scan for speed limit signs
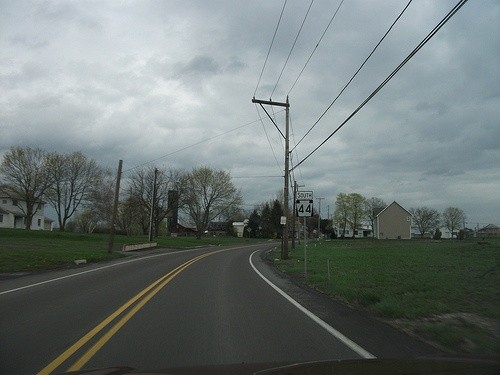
[295,200,313,217]
